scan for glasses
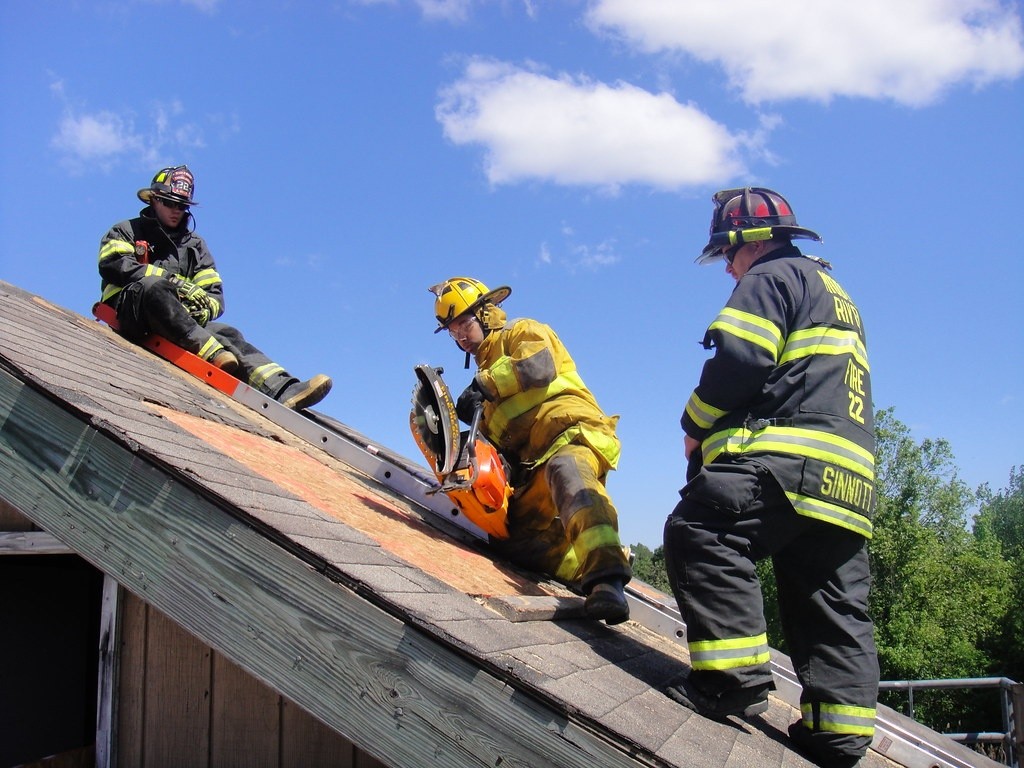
[155,197,190,211]
[449,316,477,341]
[722,240,749,266]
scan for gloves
[456,378,486,427]
[165,271,211,311]
[188,302,211,329]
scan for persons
[661,188,879,768]
[427,276,633,626]
[98,165,332,410]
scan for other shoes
[789,719,860,768]
[666,675,769,721]
[584,575,630,626]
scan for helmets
[428,276,512,335]
[694,186,823,267]
[137,164,199,206]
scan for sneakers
[209,348,239,377]
[276,374,333,413]
